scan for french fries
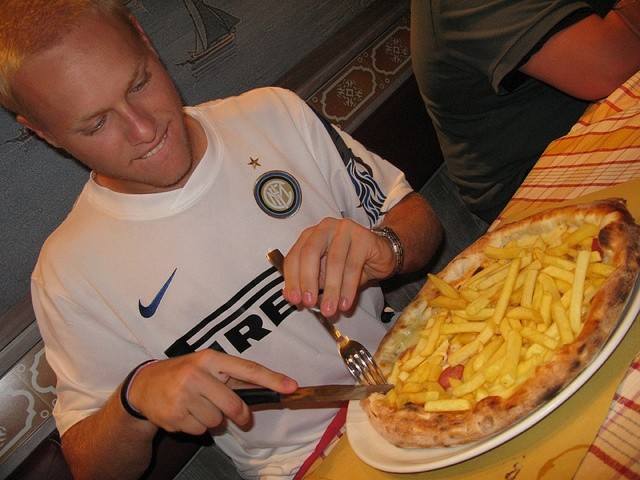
[388,221,611,411]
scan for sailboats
[175,0,242,70]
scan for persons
[0,0,447,478]
[409,1,640,228]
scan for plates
[342,284,639,475]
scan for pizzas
[359,197,637,448]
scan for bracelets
[613,1,640,37]
[368,222,406,280]
[119,356,158,422]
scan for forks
[267,249,387,388]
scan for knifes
[219,384,395,406]
